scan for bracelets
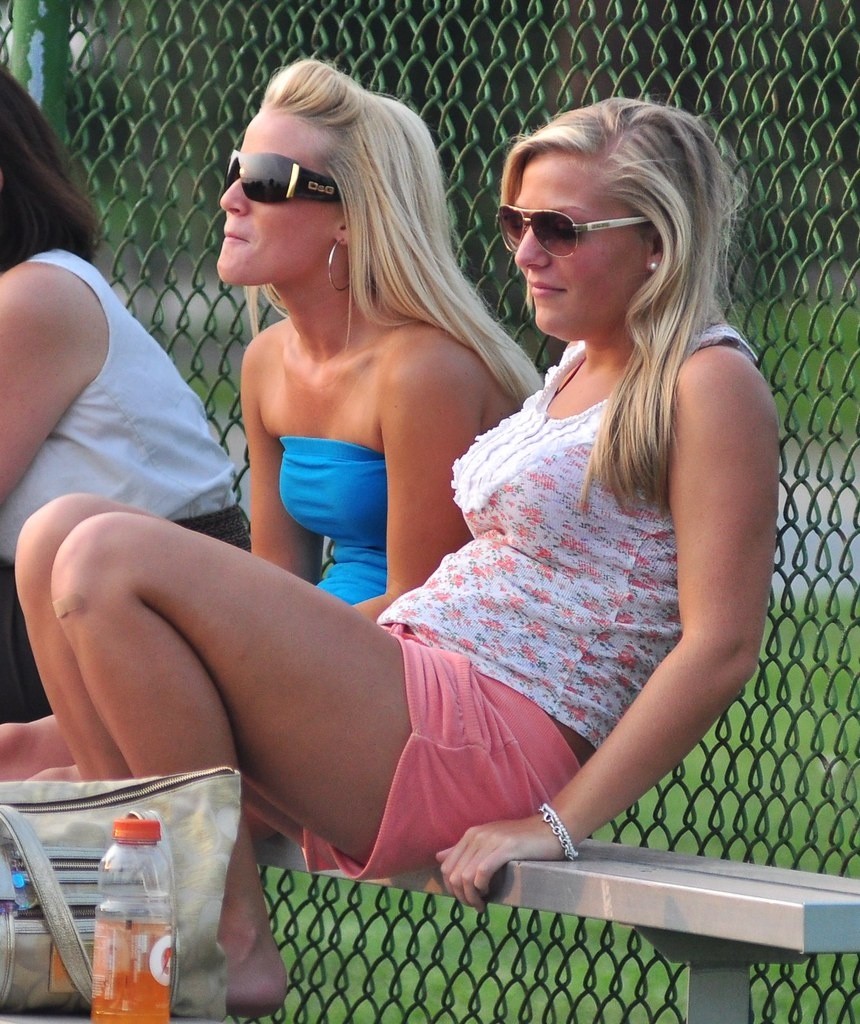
[540,803,579,860]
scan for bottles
[90,818,176,1024]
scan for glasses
[223,148,341,203]
[495,204,652,258]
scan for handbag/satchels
[0,767,241,1022]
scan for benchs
[253,822,860,1024]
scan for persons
[0,59,540,784]
[1,65,250,726]
[14,98,781,1017]
